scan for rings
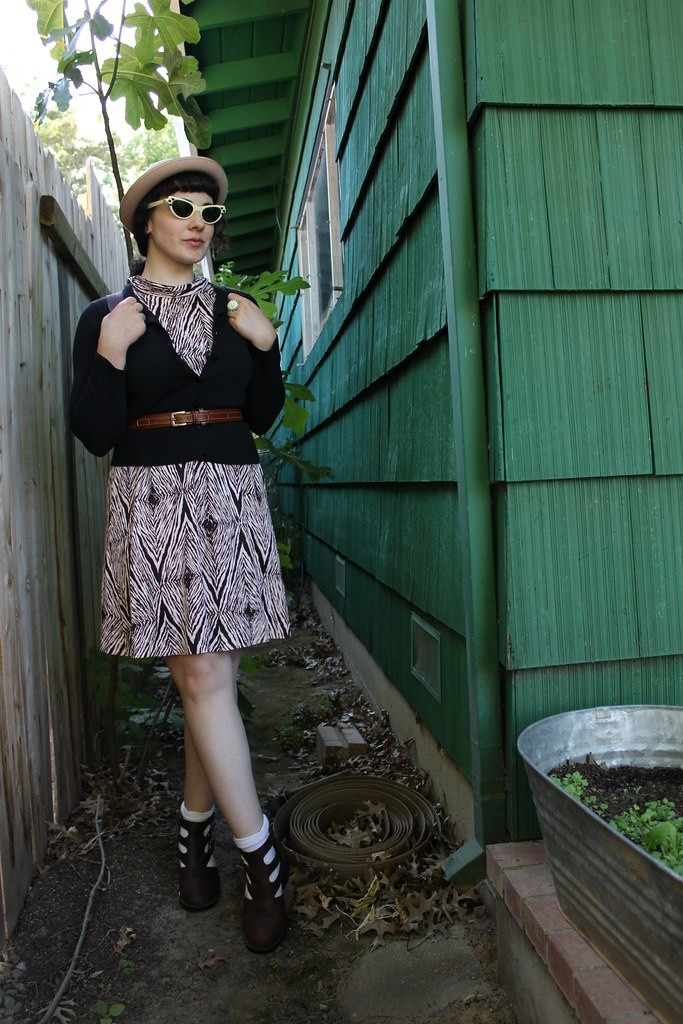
[227,299,239,311]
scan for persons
[69,156,289,950]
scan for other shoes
[235,828,291,953]
[177,813,222,911]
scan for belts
[128,408,245,429]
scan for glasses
[148,195,226,224]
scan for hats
[119,156,227,234]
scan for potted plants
[519,702,683,1024]
